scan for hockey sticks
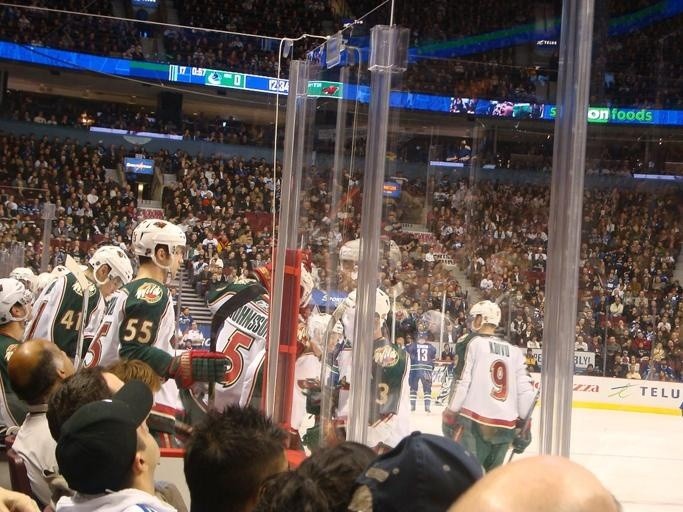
[318,292,356,452]
[61,253,89,370]
[208,285,261,411]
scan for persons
[0,0,682,512]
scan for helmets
[130,218,187,270]
[1,267,35,325]
[89,244,134,286]
[468,300,502,331]
[299,238,390,353]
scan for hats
[54,380,154,495]
[341,431,484,512]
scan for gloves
[176,350,229,388]
[252,248,312,292]
[441,408,462,437]
[512,419,533,454]
